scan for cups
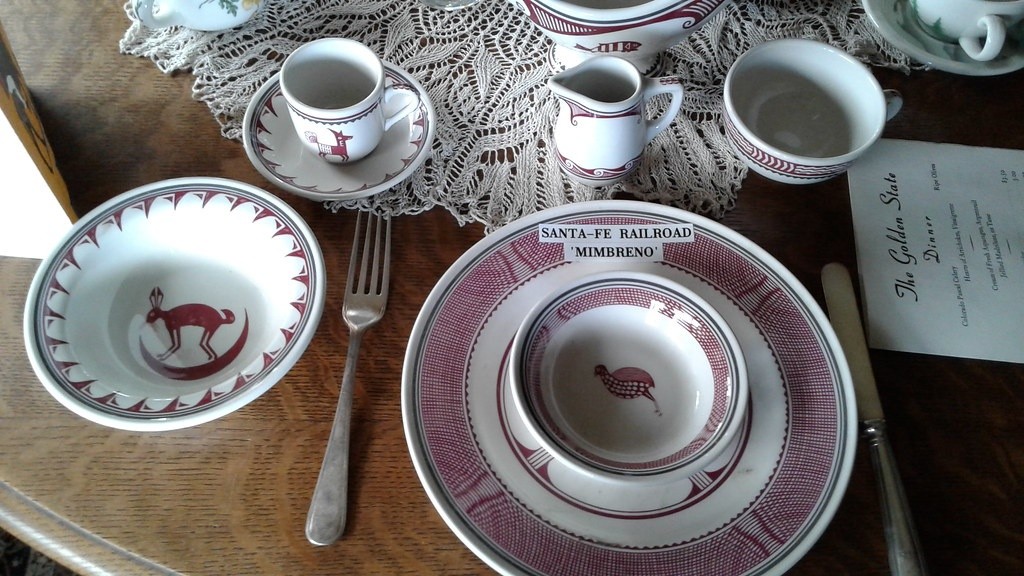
[725,38,886,184]
[901,0,1024,61]
[279,37,421,163]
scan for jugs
[548,52,684,186]
[508,0,730,92]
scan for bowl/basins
[509,268,751,483]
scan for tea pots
[132,0,271,30]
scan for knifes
[819,261,934,575]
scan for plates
[22,174,329,433]
[239,57,435,199]
[861,0,1024,75]
[401,199,858,576]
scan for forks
[301,207,392,546]
